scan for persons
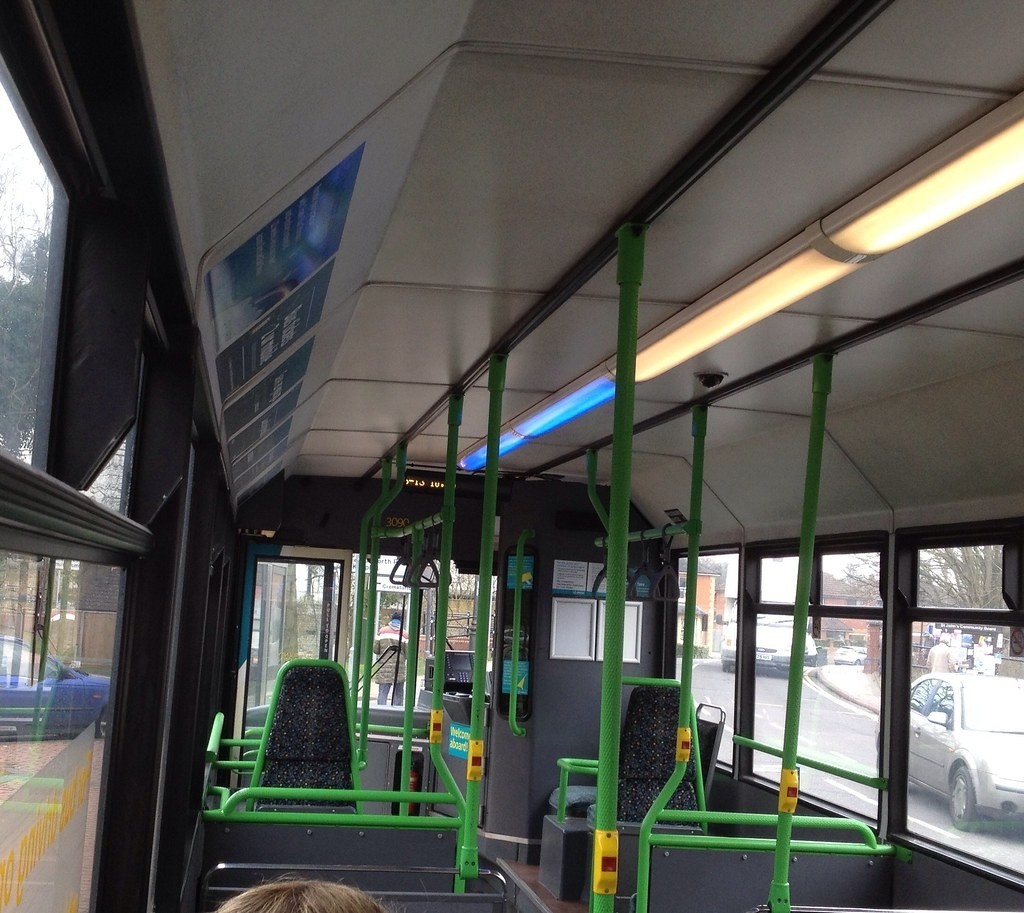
[212,870,417,913]
[373,610,409,706]
[925,632,960,673]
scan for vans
[716,601,793,678]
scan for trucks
[246,574,283,680]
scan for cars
[803,632,822,666]
[0,634,111,741]
[834,646,868,666]
[876,672,1024,832]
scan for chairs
[587,683,709,834]
[547,703,725,815]
[245,658,365,815]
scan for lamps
[452,88,1024,470]
